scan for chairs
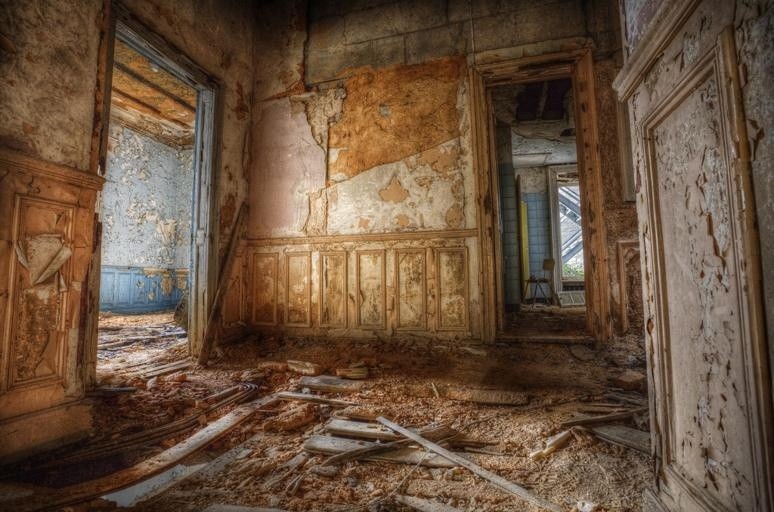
[524,257,562,308]
